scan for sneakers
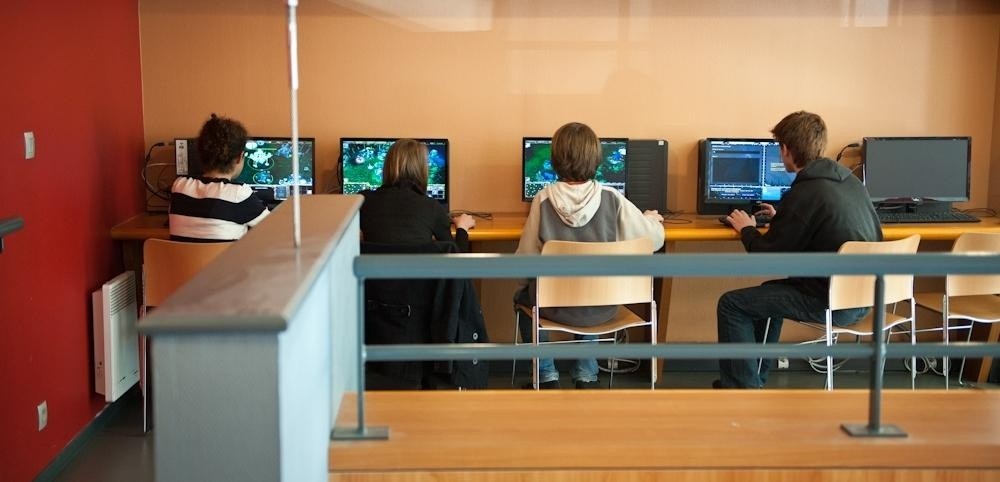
[713,379,722,388]
[522,379,562,388]
[574,375,602,389]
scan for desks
[114,207,1000,380]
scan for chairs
[754,233,920,389]
[511,239,658,389]
[911,231,1000,390]
[141,239,237,434]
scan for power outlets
[37,400,48,431]
[24,132,35,159]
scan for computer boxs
[628,138,668,214]
[697,140,750,215]
[174,137,200,177]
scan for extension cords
[607,360,620,370]
[779,358,789,369]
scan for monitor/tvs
[232,137,314,202]
[703,137,796,214]
[341,137,450,215]
[862,136,973,213]
[522,136,629,200]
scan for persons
[713,109,883,388]
[169,112,271,243]
[357,138,492,389]
[513,121,665,389]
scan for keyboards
[877,213,982,223]
[718,216,772,228]
